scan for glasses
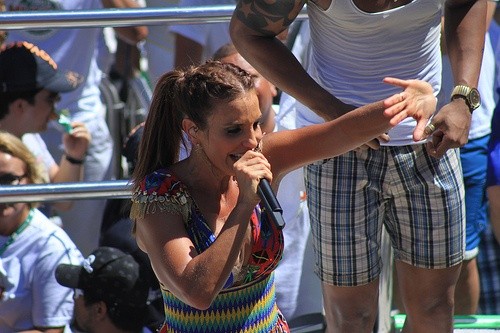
[0,172,28,185]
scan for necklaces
[198,149,233,216]
[0,208,34,257]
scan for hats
[55,246,149,306]
[0,41,84,91]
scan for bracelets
[64,149,87,165]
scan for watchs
[450,85,481,110]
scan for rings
[427,123,436,132]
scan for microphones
[257,178,286,230]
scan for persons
[55,247,148,332]
[441,0,500,315]
[0,0,149,259]
[100,122,167,333]
[0,132,85,333]
[229,0,488,333]
[211,43,323,331]
[0,41,91,228]
[166,0,289,72]
[121,60,437,333]
